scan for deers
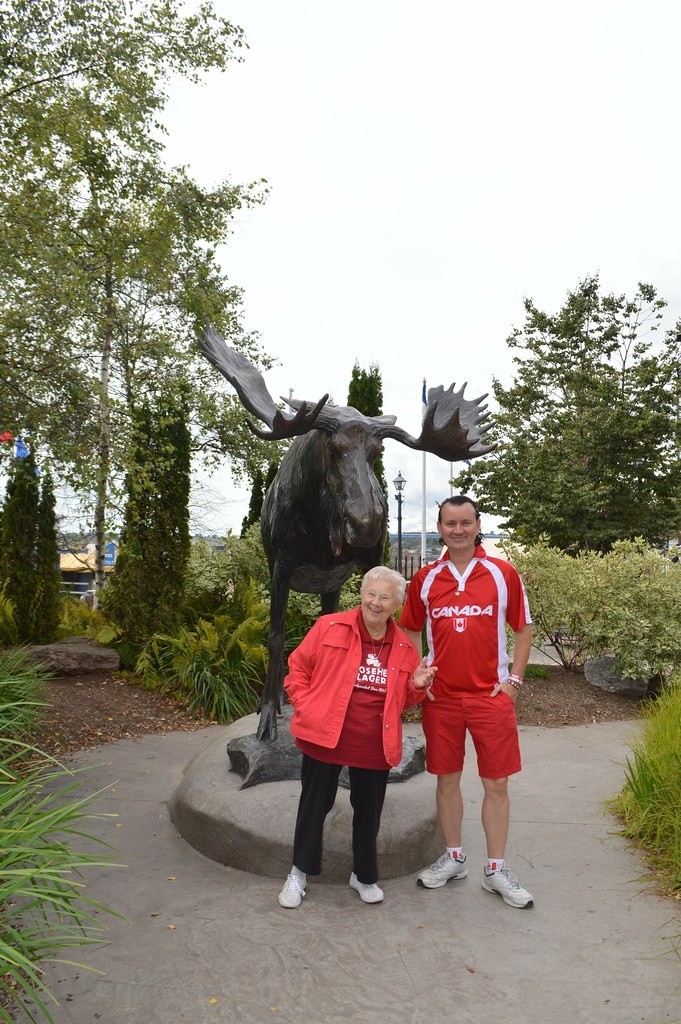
[192,318,500,741]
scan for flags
[464,460,471,466]
[422,383,428,416]
[17,436,40,475]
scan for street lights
[393,470,406,574]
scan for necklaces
[372,637,384,659]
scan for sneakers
[481,866,535,910]
[349,871,385,904]
[417,853,470,889]
[278,873,308,909]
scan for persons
[399,495,534,909]
[507,673,524,690]
[278,565,438,908]
